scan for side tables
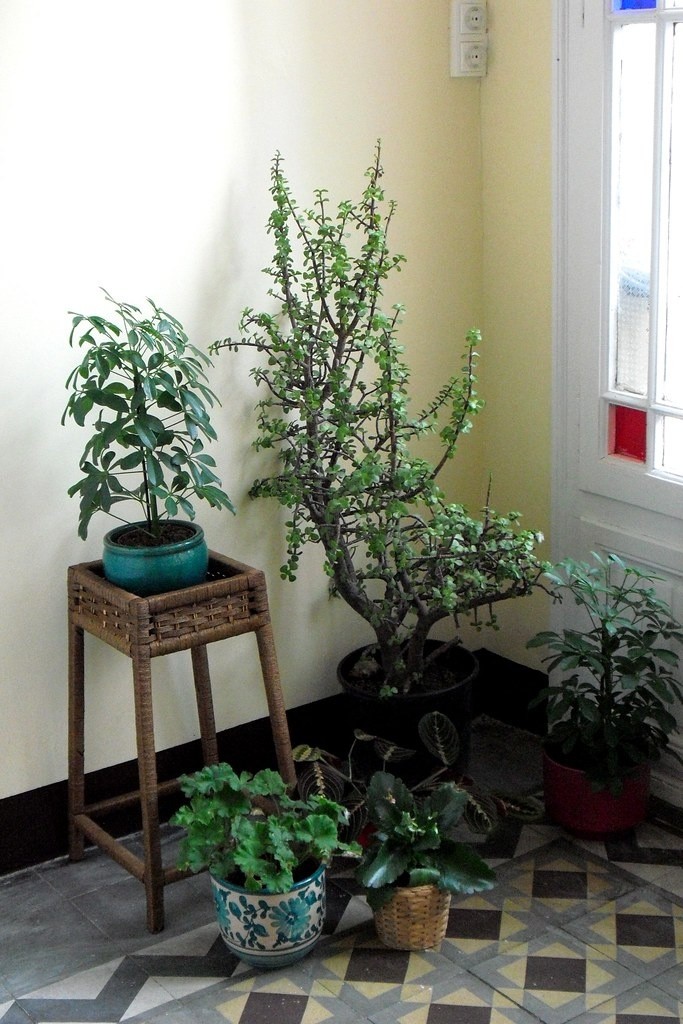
[67,552,301,928]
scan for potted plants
[209,136,566,782]
[354,774,500,950]
[57,278,236,593]
[528,547,683,830]
[171,763,361,968]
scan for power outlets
[450,1,488,81]
